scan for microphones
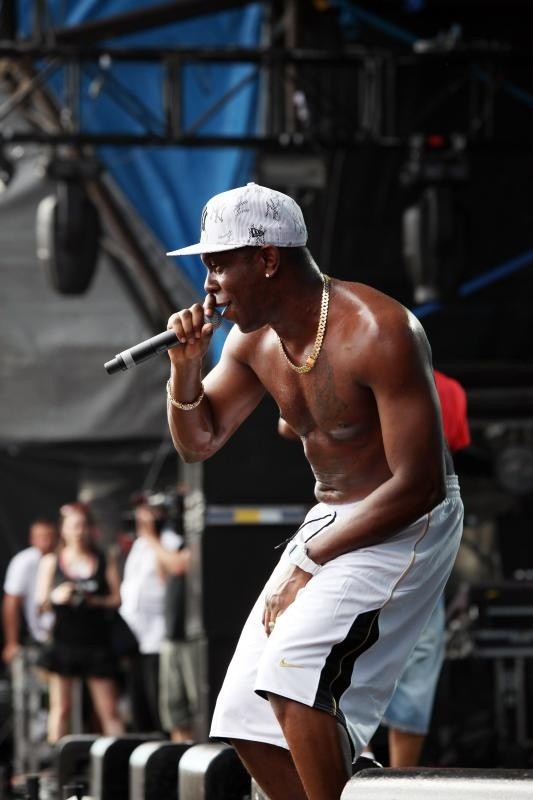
[103,309,223,378]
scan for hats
[165,181,308,256]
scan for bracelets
[166,378,204,411]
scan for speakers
[182,391,318,747]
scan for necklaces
[276,274,330,374]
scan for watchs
[287,542,322,575]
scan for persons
[0,490,195,747]
[165,182,464,800]
[346,328,470,774]
